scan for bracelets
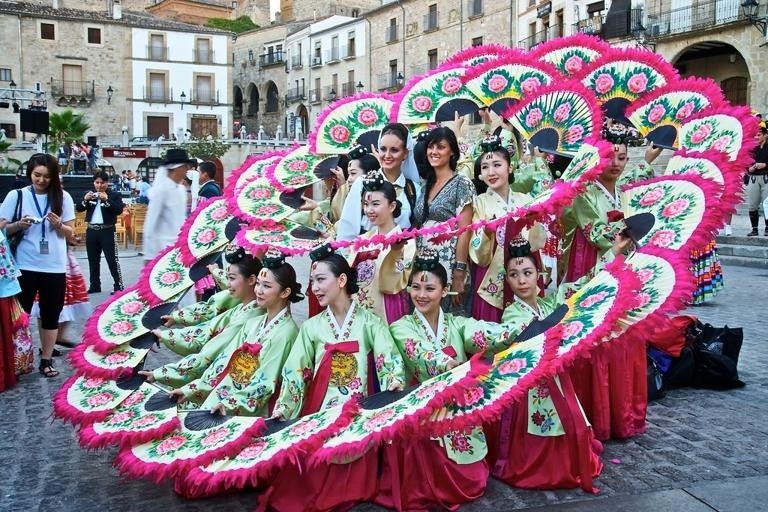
[56,222,63,230]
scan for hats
[160,148,198,166]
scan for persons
[335,172,417,325]
[160,262,244,326]
[755,114,765,127]
[0,152,76,376]
[164,259,299,499]
[156,127,258,141]
[272,244,406,512]
[138,243,261,391]
[76,170,124,293]
[57,143,98,180]
[335,124,422,259]
[286,155,349,225]
[411,128,472,314]
[747,126,768,238]
[724,210,732,236]
[119,169,152,206]
[389,246,538,512]
[299,155,380,224]
[568,132,672,444]
[189,160,223,201]
[502,227,634,489]
[141,150,191,264]
[467,136,569,324]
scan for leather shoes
[39,349,62,358]
[746,229,755,240]
[111,290,119,294]
[86,288,103,294]
[57,339,78,349]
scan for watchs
[454,261,467,271]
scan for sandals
[39,359,60,377]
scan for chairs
[115,215,127,247]
[74,210,87,243]
[130,206,147,250]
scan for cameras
[24,213,47,225]
[91,192,99,198]
